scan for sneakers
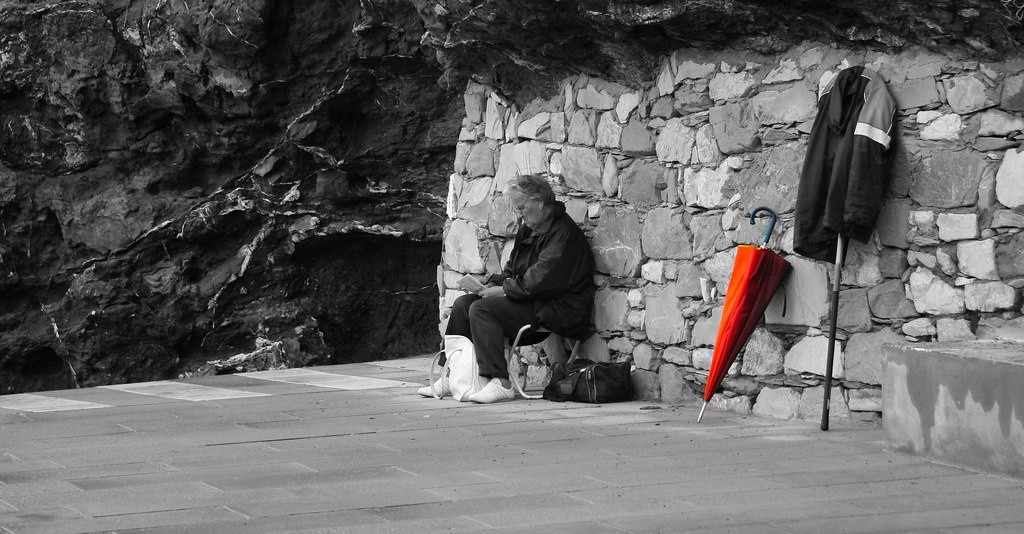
[469,378,515,404]
[418,376,451,397]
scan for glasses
[513,199,528,216]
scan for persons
[417,174,595,404]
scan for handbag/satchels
[429,335,491,401]
[543,357,635,404]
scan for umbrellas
[695,205,794,425]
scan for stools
[503,323,580,399]
